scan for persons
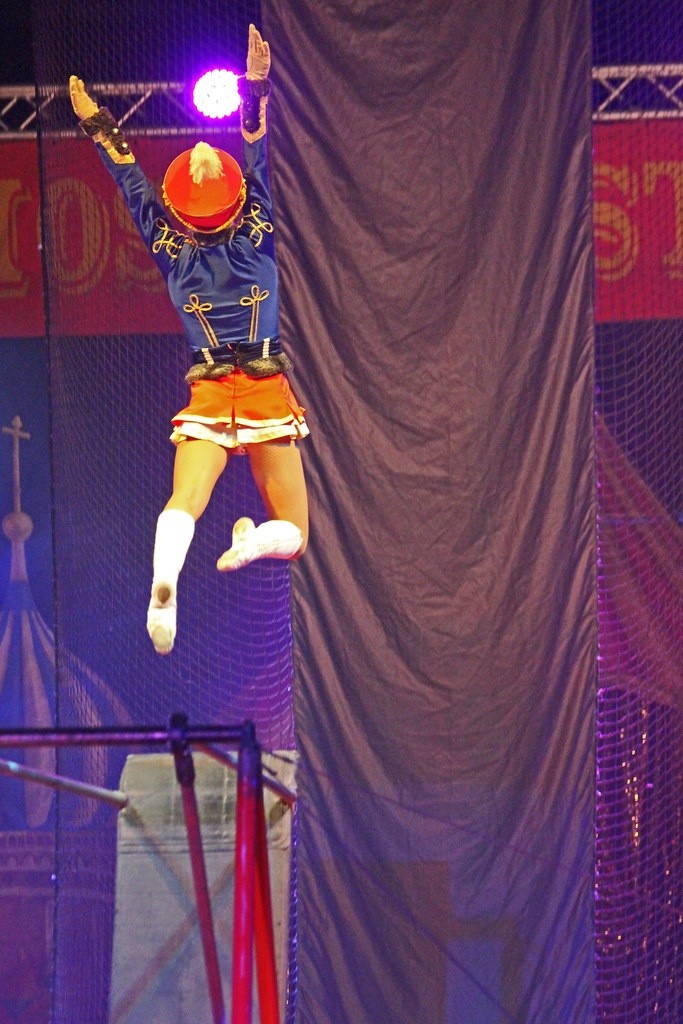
[70,23,310,654]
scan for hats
[163,142,245,235]
